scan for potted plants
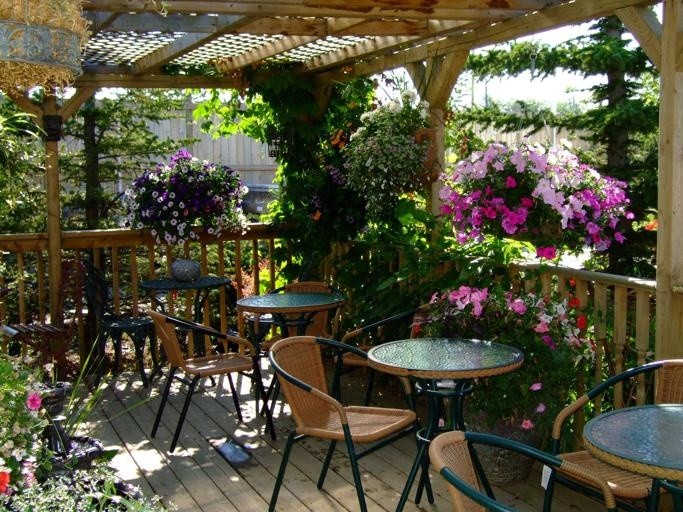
[40,336,157,477]
[0,472,179,512]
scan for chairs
[8,260,83,380]
[429,430,616,512]
[255,281,344,435]
[270,336,435,512]
[85,267,163,388]
[148,309,276,453]
[334,303,433,411]
[542,359,683,512]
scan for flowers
[344,90,432,223]
[125,150,248,247]
[438,141,634,258]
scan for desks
[139,276,230,390]
[583,403,683,512]
[237,293,344,433]
[368,337,523,512]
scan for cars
[241,183,285,222]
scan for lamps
[0,0,93,98]
